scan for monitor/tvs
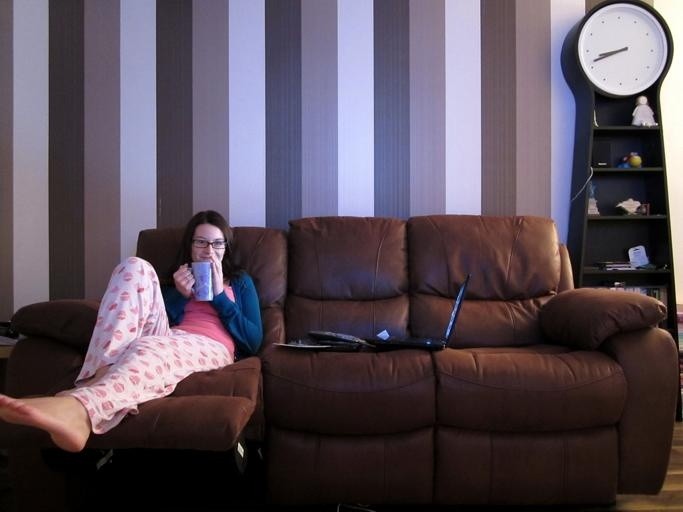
[597,259,635,270]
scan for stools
[33,356,264,512]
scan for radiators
[319,340,360,350]
[308,330,360,343]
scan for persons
[1,210,263,452]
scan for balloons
[577,2,669,99]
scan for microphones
[33,356,264,512]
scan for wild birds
[376,280,467,351]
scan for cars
[184,261,214,302]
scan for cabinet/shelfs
[561,0,683,422]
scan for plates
[561,0,683,422]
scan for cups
[192,239,228,250]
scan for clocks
[577,2,669,99]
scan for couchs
[0,216,680,512]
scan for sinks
[0,244,680,512]
